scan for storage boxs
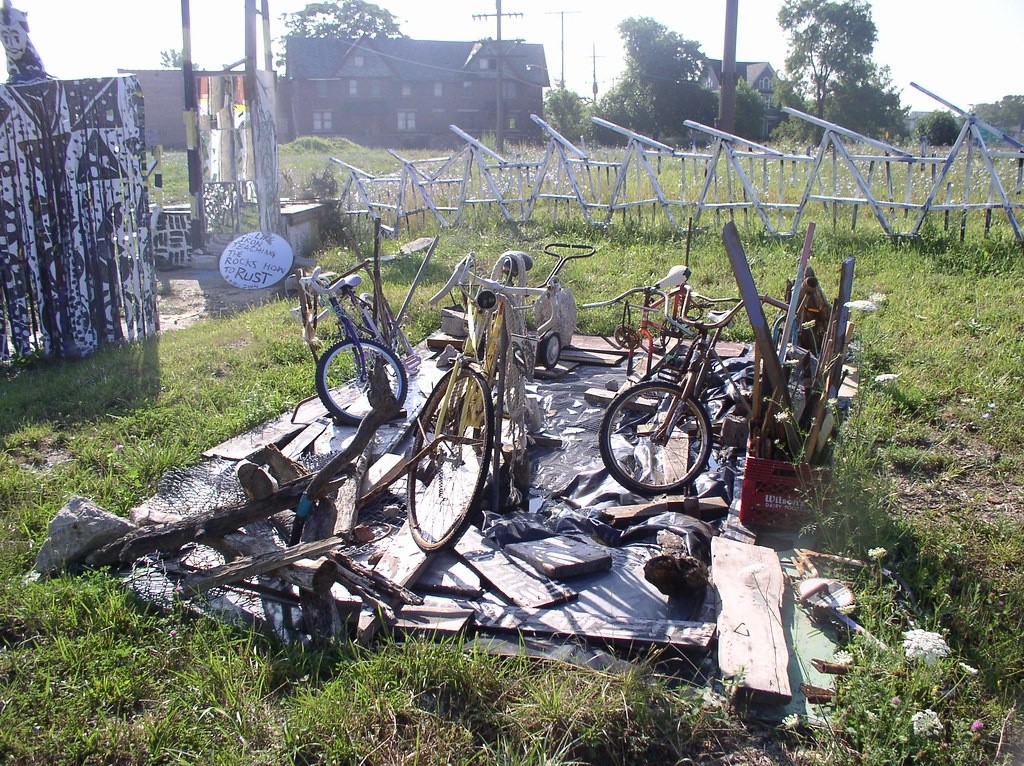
[741,440,832,530]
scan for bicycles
[298,267,422,427]
[405,248,560,553]
[599,284,789,494]
